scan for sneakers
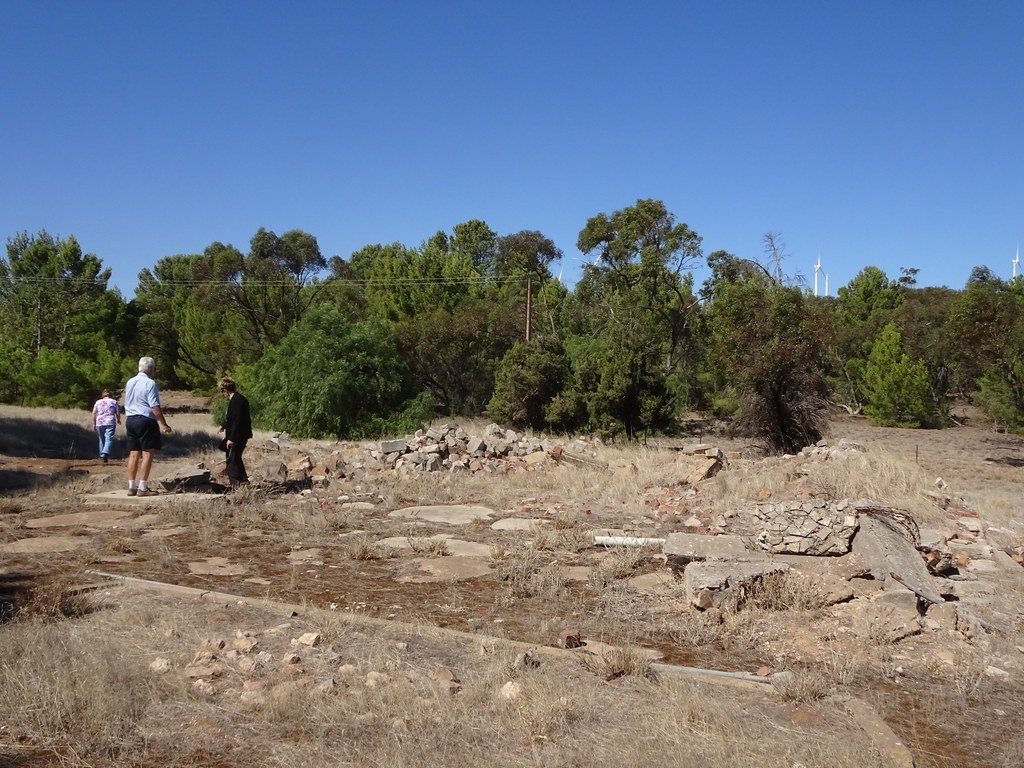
[137,487,159,497]
[127,488,138,496]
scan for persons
[216,377,253,487]
[93,389,123,464]
[124,357,173,497]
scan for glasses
[221,390,224,393]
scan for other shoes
[99,454,108,463]
